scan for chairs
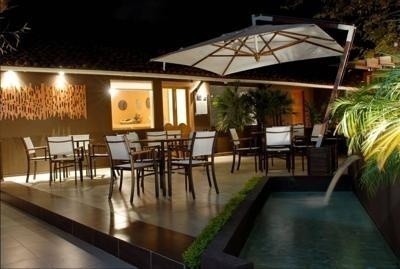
[21,129,219,204]
[229,123,328,175]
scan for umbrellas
[150,15,357,147]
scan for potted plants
[212,79,255,155]
